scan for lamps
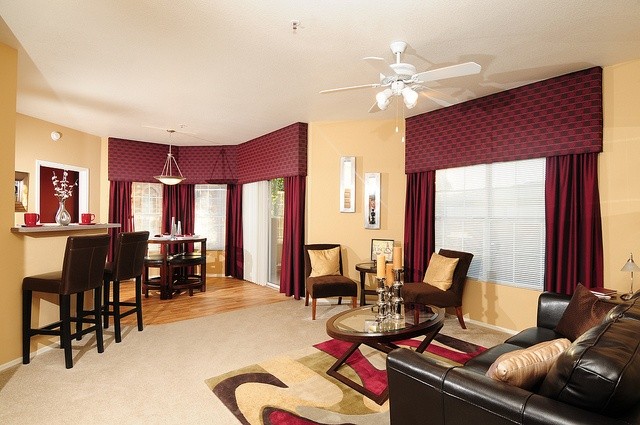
[620,253,640,301]
[153,130,187,186]
[376,80,419,111]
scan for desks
[355,262,407,305]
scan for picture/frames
[371,239,394,261]
[340,156,356,213]
[15,171,29,212]
[363,173,381,230]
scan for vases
[54,202,71,226]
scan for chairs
[103,231,149,343]
[399,249,474,329]
[22,235,111,369]
[304,244,357,320]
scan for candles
[386,264,394,280]
[376,251,386,278]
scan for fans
[320,42,483,115]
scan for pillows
[307,246,341,278]
[422,252,460,292]
[484,338,572,389]
[552,282,617,341]
[600,296,640,323]
[537,319,639,416]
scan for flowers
[51,169,79,202]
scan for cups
[81,214,95,223]
[24,213,39,225]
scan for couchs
[386,293,639,425]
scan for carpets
[203,331,488,424]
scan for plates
[22,225,42,227]
[79,223,96,225]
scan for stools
[144,253,205,300]
